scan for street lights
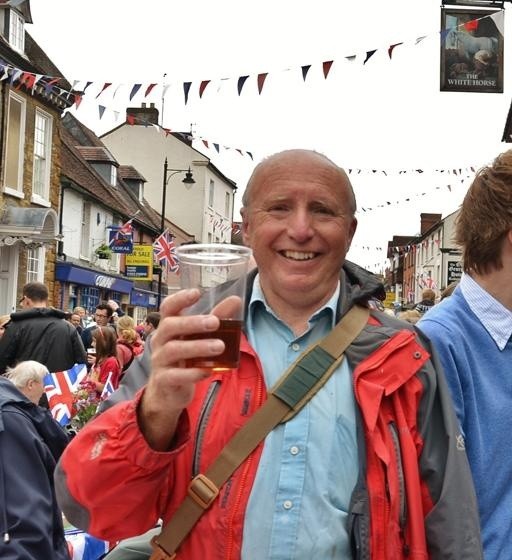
[159,155,196,309]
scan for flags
[113,215,135,241]
[152,229,181,272]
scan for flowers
[72,377,105,424]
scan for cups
[173,241,253,374]
[87,347,97,364]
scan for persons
[414,148,511,559]
[51,147,484,560]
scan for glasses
[19,296,25,306]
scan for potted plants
[94,244,113,260]
